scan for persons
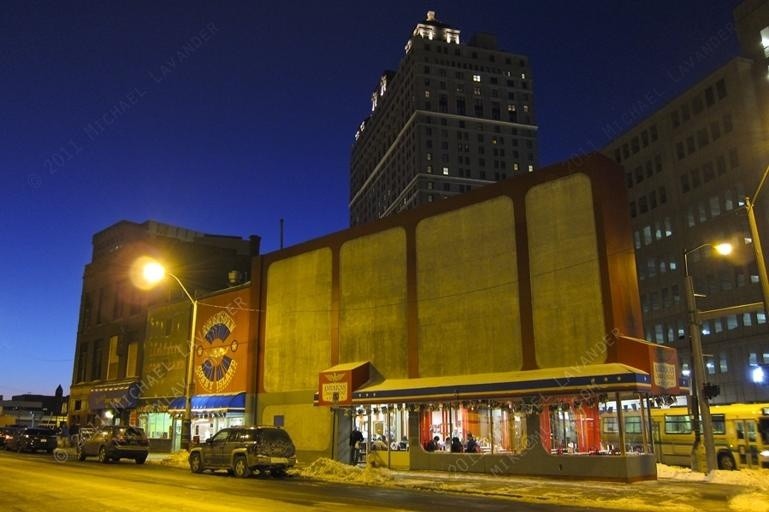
[451,437,462,453]
[372,435,389,450]
[441,438,451,452]
[464,432,477,453]
[348,426,364,466]
[426,436,439,450]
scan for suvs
[188,424,297,477]
[0,420,60,454]
[74,423,151,468]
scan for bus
[599,403,769,473]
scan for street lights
[142,260,198,458]
[682,241,736,474]
